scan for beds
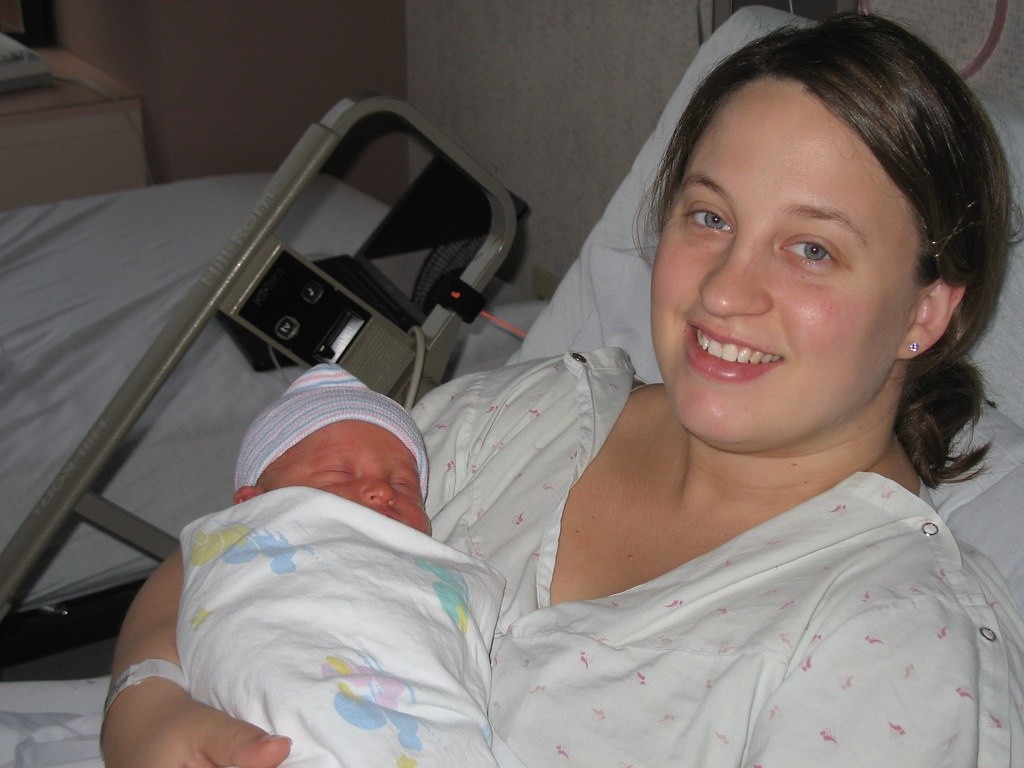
[0,96,549,622]
[0,6,1024,768]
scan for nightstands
[0,47,150,209]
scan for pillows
[570,243,1024,585]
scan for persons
[1,17,1024,768]
[177,360,496,766]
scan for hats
[235,363,428,504]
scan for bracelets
[97,658,189,758]
